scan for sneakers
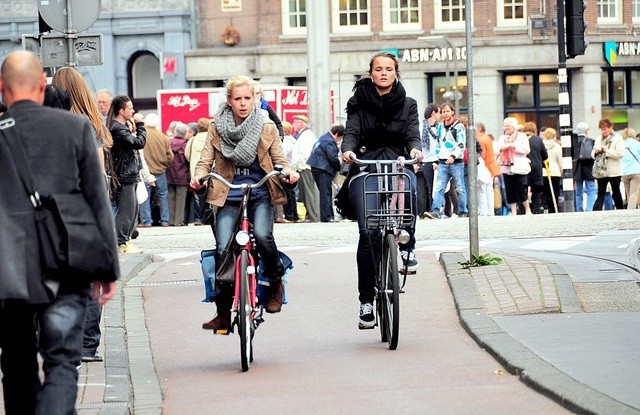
[359,303,376,327]
[424,212,439,219]
[82,350,103,361]
[401,250,418,270]
[120,238,143,254]
[457,213,467,217]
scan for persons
[306,123,345,223]
[635,133,640,142]
[184,116,212,225]
[572,121,596,212]
[538,127,546,138]
[186,122,199,140]
[0,50,120,415]
[139,112,174,227]
[165,123,190,225]
[591,118,625,210]
[474,120,500,216]
[165,120,181,137]
[252,79,284,144]
[494,115,532,216]
[188,75,300,329]
[458,117,482,188]
[106,94,147,254]
[541,126,565,213]
[418,104,442,218]
[49,65,113,362]
[95,89,112,126]
[620,127,640,210]
[39,84,73,112]
[280,120,300,224]
[424,102,467,220]
[521,121,548,214]
[339,50,425,329]
[290,115,320,223]
[124,117,157,241]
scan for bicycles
[191,164,294,371]
[343,153,431,349]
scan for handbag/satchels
[216,226,237,295]
[594,154,608,177]
[494,185,502,209]
[578,138,593,159]
[35,193,118,284]
[201,250,293,304]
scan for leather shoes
[266,278,283,313]
[202,311,231,329]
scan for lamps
[246,54,256,71]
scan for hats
[197,118,209,129]
[293,116,308,125]
